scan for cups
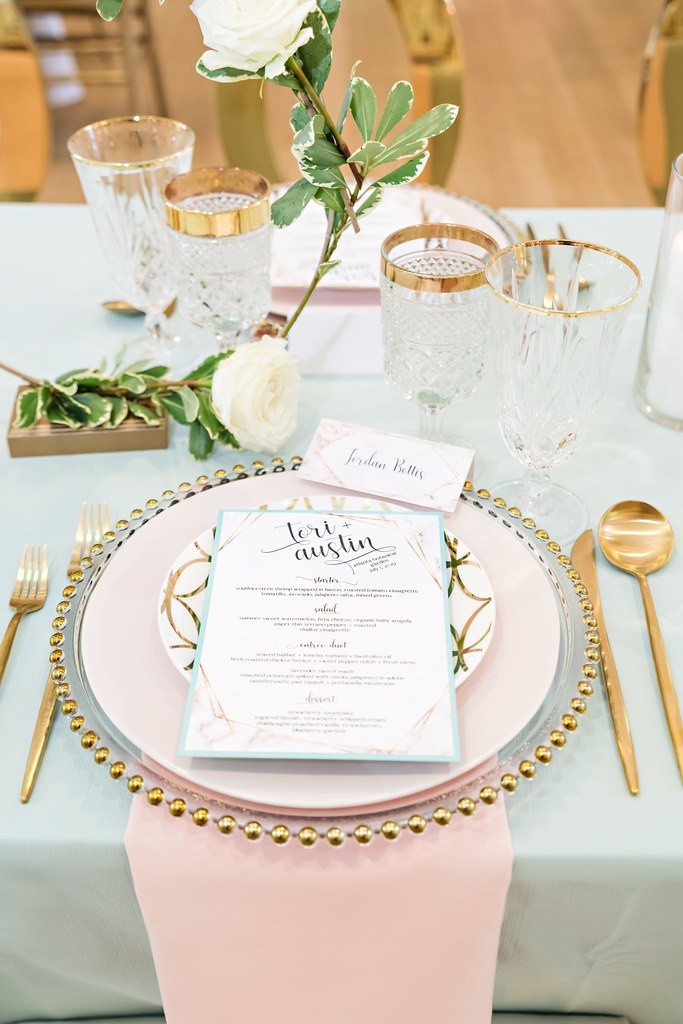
[633,152,683,433]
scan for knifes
[572,530,641,795]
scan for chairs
[2,0,683,210]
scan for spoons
[102,300,143,317]
[599,500,683,785]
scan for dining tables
[0,198,683,1024]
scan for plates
[49,457,601,851]
[270,181,526,299]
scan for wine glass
[485,239,642,546]
[379,223,501,439]
[68,115,196,371]
[158,166,272,352]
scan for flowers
[0,0,471,455]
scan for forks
[0,543,49,682]
[21,500,111,802]
